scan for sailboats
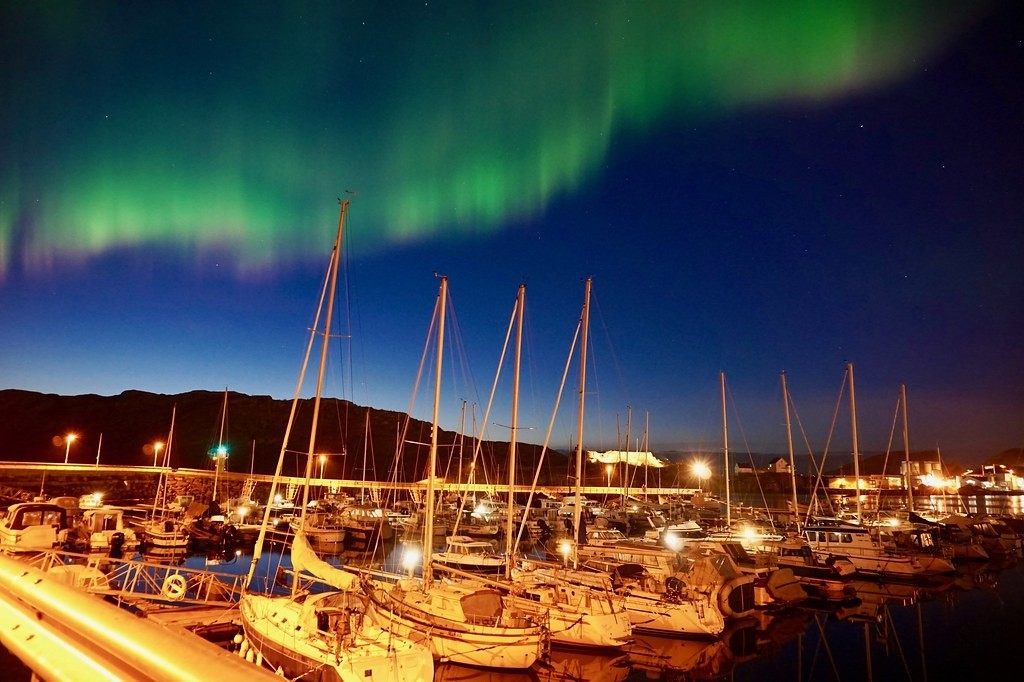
[0,189,1024,680]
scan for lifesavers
[162,574,186,598]
